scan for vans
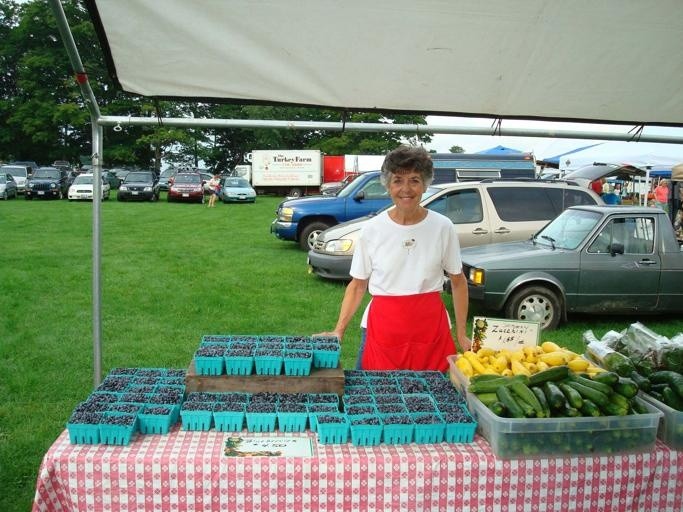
[302,161,646,285]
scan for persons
[312,144,474,370]
[207,176,221,207]
[589,177,633,205]
[649,177,683,240]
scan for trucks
[270,153,537,258]
[229,147,324,197]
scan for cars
[0,155,127,193]
[219,176,256,203]
[538,173,559,181]
[158,177,169,191]
[166,172,206,202]
[116,171,158,202]
[67,174,110,201]
[200,172,221,190]
[0,174,17,200]
[320,174,357,193]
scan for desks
[30,416,683,512]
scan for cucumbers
[467,330,683,459]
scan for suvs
[21,167,67,200]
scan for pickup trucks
[444,204,683,327]
